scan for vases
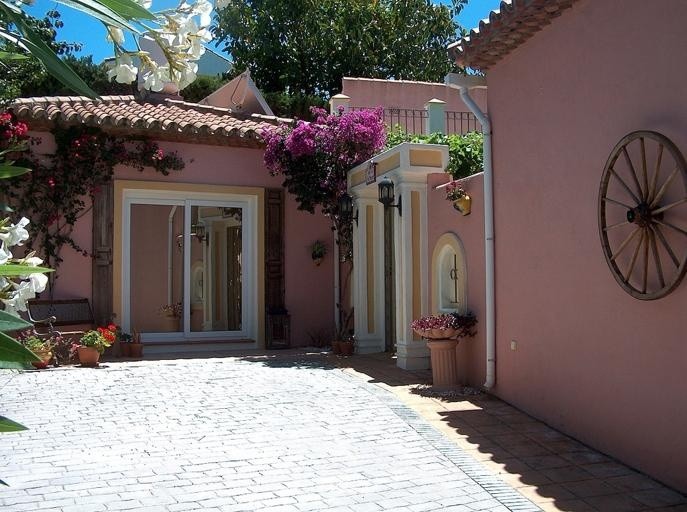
[164,313,181,332]
[76,344,101,367]
[119,342,145,358]
[30,347,53,369]
[411,327,465,392]
[452,194,472,216]
[313,257,324,267]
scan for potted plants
[331,302,355,356]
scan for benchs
[25,297,97,343]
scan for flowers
[313,240,330,257]
[159,298,183,321]
[444,181,466,201]
[78,323,117,356]
[408,309,479,340]
[17,329,49,353]
[118,327,141,344]
